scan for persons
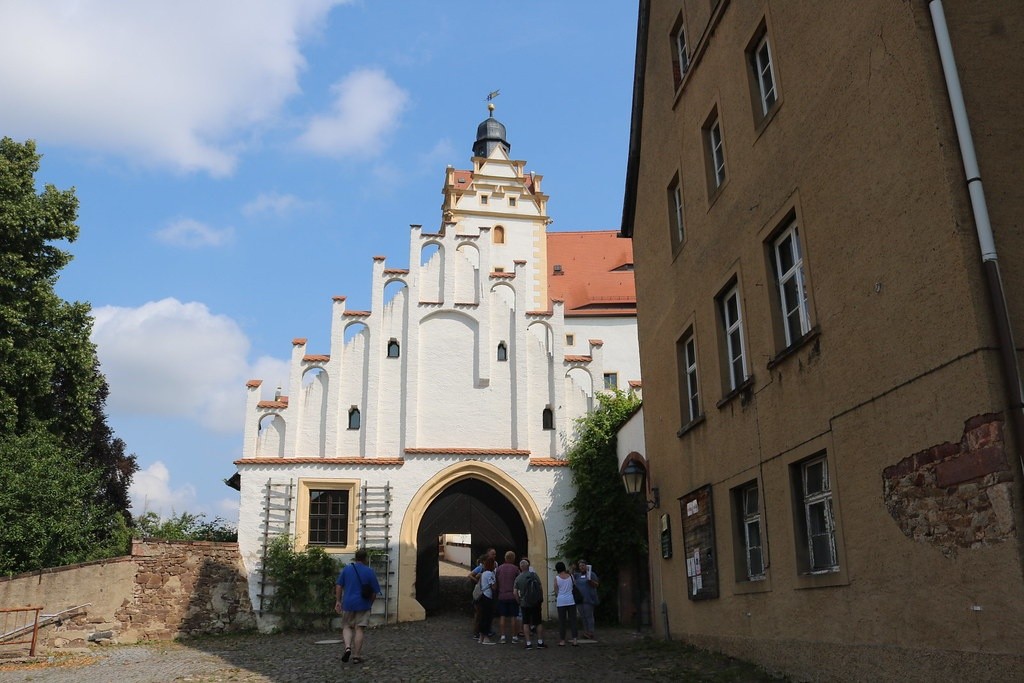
[334,547,381,664]
[513,560,548,650]
[555,562,578,647]
[468,549,536,645]
[574,560,601,640]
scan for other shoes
[523,644,533,650]
[584,632,594,639]
[572,642,577,646]
[512,639,522,643]
[537,642,548,648]
[472,634,479,639]
[497,638,506,643]
[482,639,496,644]
[478,639,482,643]
[488,631,496,636]
[557,642,566,646]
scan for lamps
[618,460,659,518]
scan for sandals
[342,647,352,662]
[353,657,364,664]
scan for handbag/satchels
[569,573,584,604]
[473,578,483,600]
[361,584,372,598]
[590,586,598,603]
